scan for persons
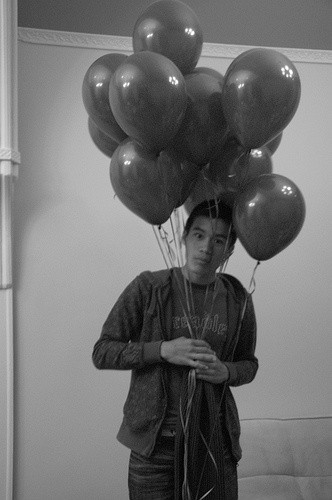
[92,201,258,500]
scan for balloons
[82,1,307,260]
